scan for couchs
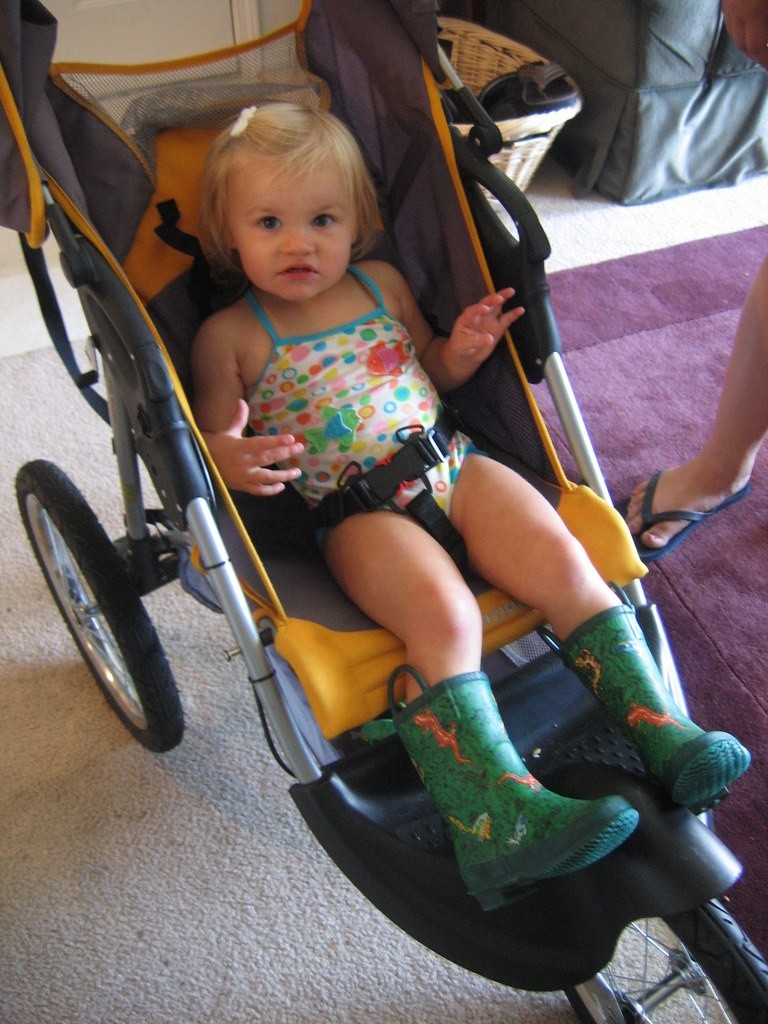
[439,0,768,207]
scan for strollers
[0,1,768,1024]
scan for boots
[536,581,751,807]
[387,664,638,911]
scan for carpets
[530,224,768,959]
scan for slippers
[519,58,577,111]
[613,471,751,561]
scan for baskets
[433,16,584,213]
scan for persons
[616,0,766,559]
[186,94,750,911]
